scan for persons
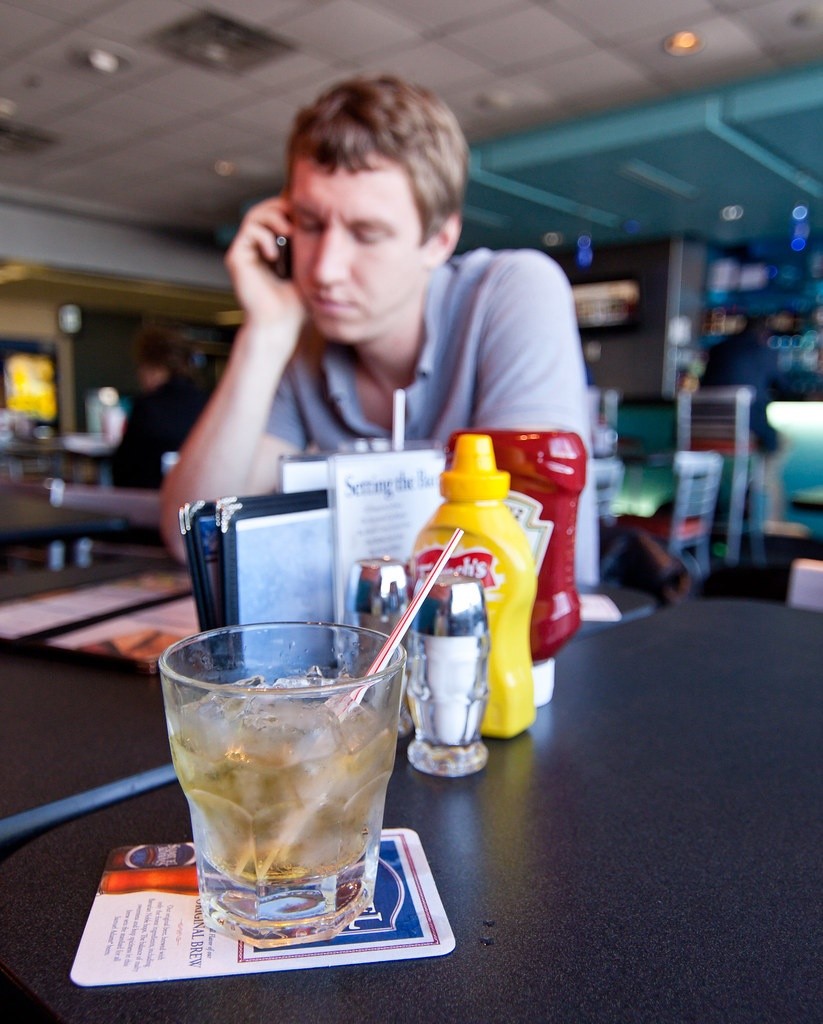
[699,320,793,472]
[108,327,207,490]
[159,71,602,587]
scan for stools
[676,387,767,565]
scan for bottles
[412,433,538,741]
[440,428,589,709]
[333,556,491,780]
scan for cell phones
[273,233,291,279]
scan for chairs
[601,445,724,593]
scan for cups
[156,619,407,952]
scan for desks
[0,566,823,1024]
